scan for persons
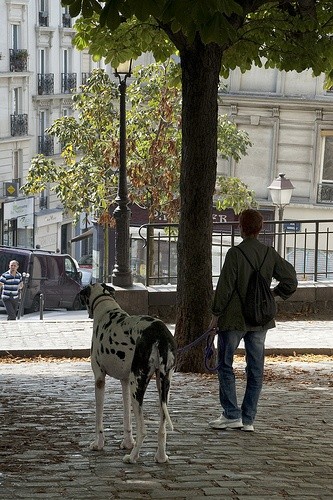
[209,209,298,431]
[0,260,23,321]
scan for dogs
[78,281,177,464]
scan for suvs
[0,244,86,313]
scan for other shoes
[240,424,254,431]
[208,413,243,429]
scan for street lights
[109,58,139,287]
[267,172,296,259]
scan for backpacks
[233,245,278,327]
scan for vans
[127,226,244,287]
